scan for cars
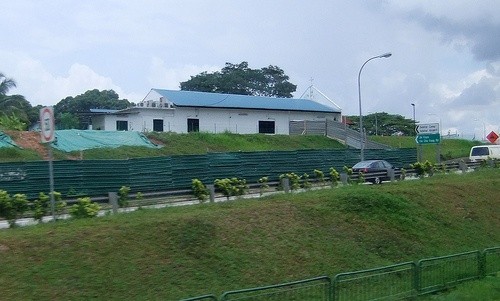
[349,160,406,185]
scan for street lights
[411,103,416,135]
[358,51,392,161]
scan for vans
[468,145,500,163]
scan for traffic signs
[414,133,440,143]
[414,122,439,134]
